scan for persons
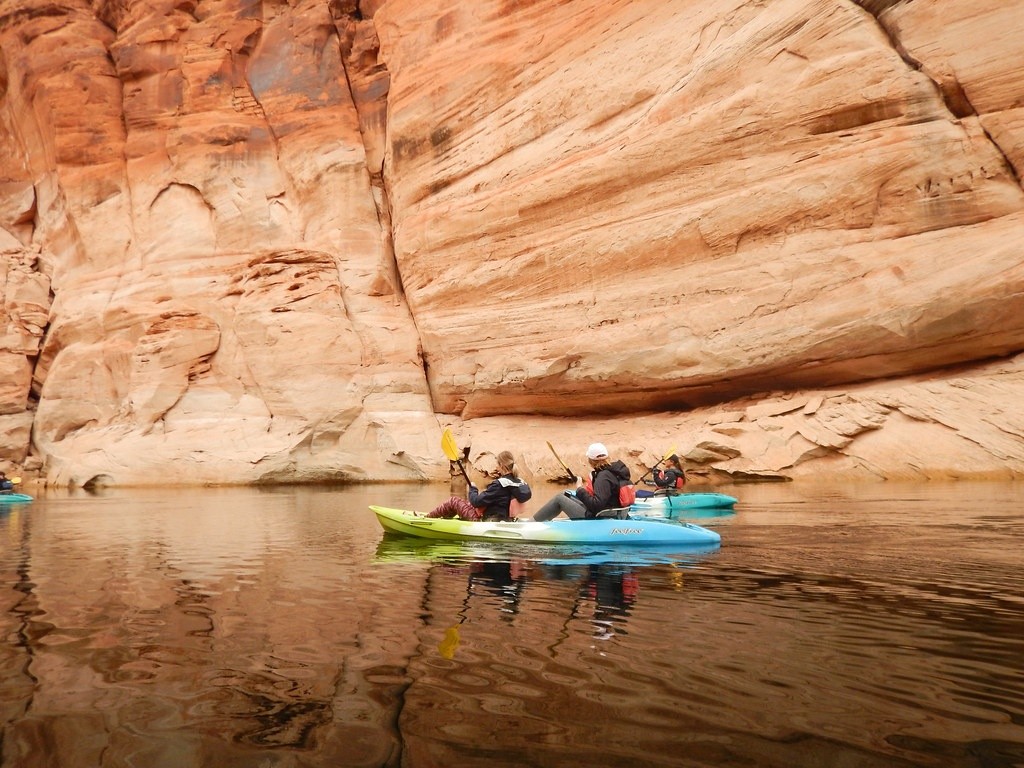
[0,472,13,489]
[403,451,532,522]
[514,443,635,523]
[636,454,686,497]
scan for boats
[0,493,34,501]
[565,489,739,508]
[366,503,722,545]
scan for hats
[586,443,609,460]
[669,454,679,463]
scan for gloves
[640,477,646,483]
[652,468,659,479]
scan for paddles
[11,477,21,484]
[441,428,473,487]
[634,445,678,485]
[546,439,578,484]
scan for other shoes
[414,510,419,516]
